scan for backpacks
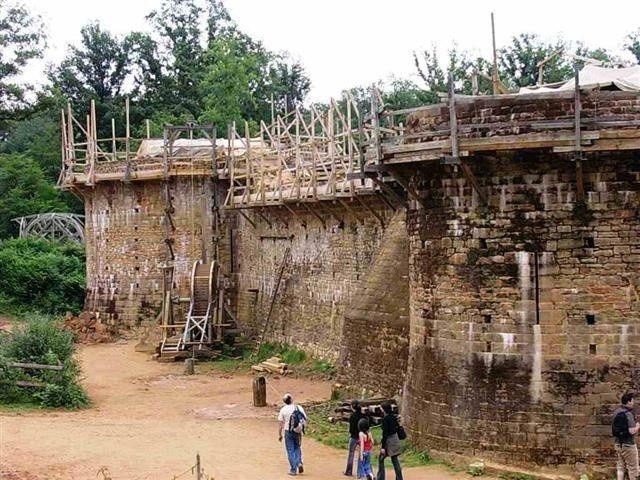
[612,412,629,439]
[288,403,306,434]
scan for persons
[343,400,365,475]
[376,405,403,480]
[612,393,640,480]
[277,394,307,476]
[358,418,375,479]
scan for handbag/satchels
[397,426,406,439]
